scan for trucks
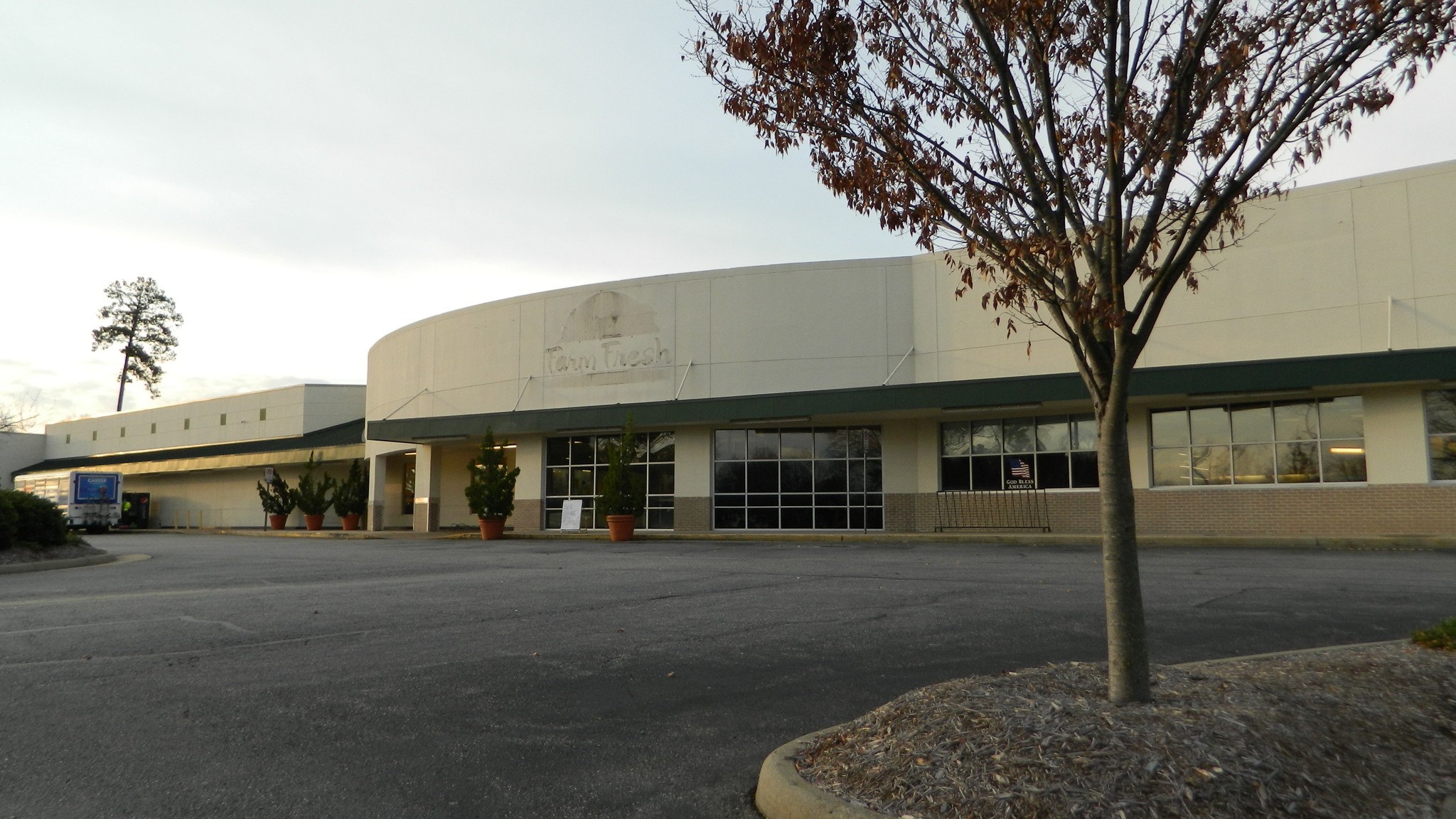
[14,470,123,535]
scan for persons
[121,496,148,527]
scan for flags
[1007,457,1030,479]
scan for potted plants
[332,458,369,530]
[257,466,296,530]
[464,426,521,541]
[594,413,647,542]
[292,450,333,531]
